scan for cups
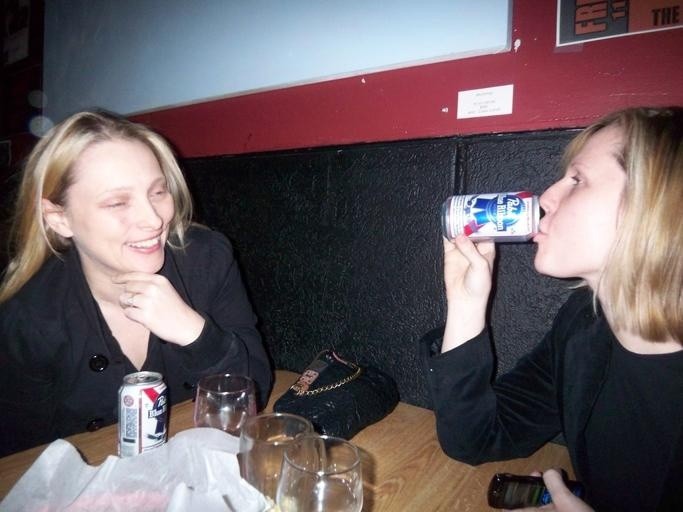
[195,371,366,512]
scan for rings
[124,291,139,308]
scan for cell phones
[488,472,582,510]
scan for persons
[0,109,275,453]
[429,103,683,512]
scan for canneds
[116,371,168,456]
[440,191,539,244]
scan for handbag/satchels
[271,347,403,449]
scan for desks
[2,363,581,512]
